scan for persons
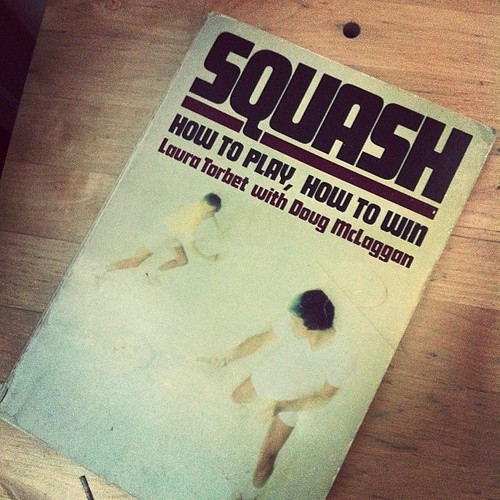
[209,289,349,500]
[94,192,223,289]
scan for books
[1,11,499,500]
